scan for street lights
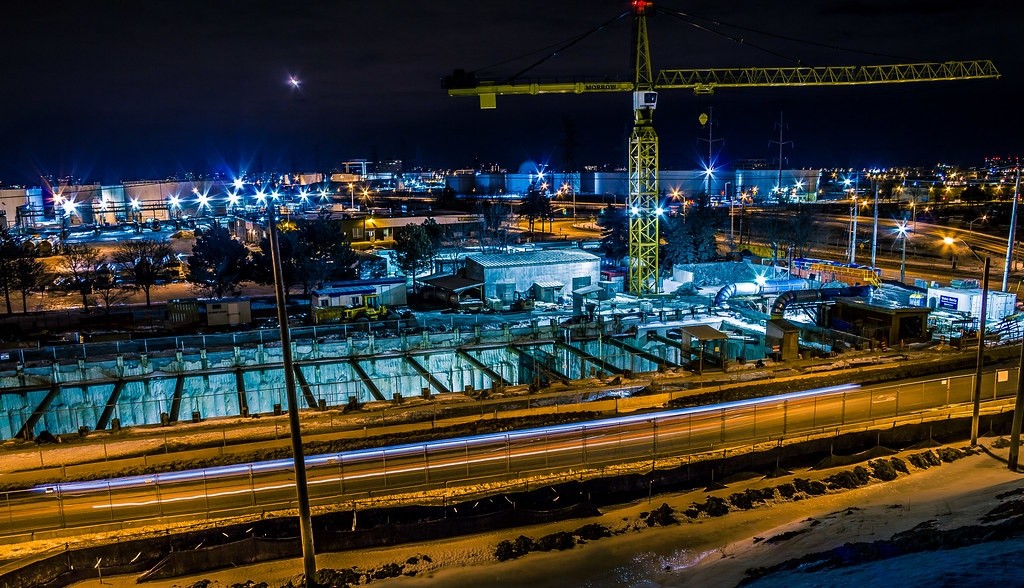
[897,224,907,286]
[941,236,990,448]
[673,191,685,225]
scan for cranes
[448,0,1003,298]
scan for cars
[51,252,196,291]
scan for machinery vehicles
[344,295,389,323]
